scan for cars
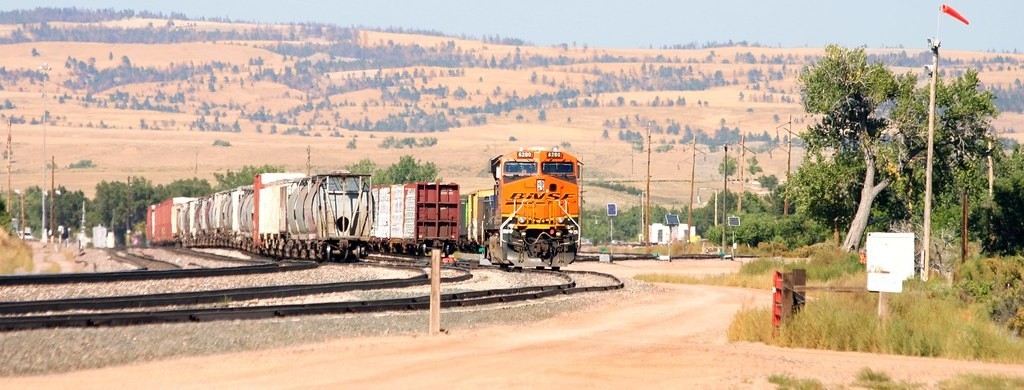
[19,231,32,239]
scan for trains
[368,182,460,256]
[458,147,581,271]
[146,170,376,262]
